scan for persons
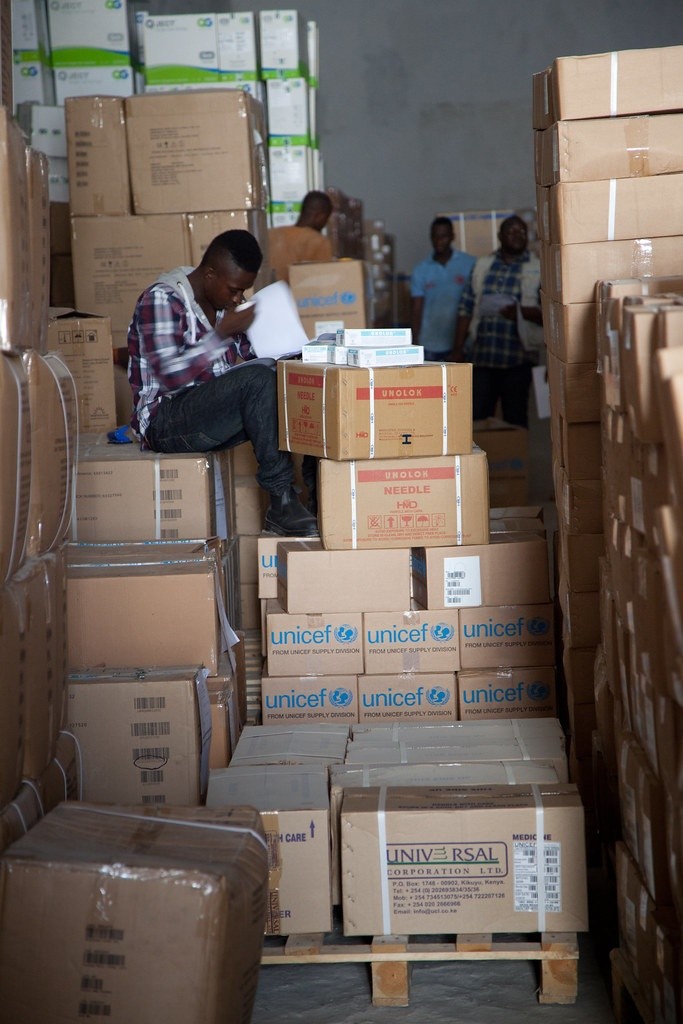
[258,190,333,284]
[127,229,319,538]
[410,215,541,431]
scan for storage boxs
[1,1,683,1022]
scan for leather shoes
[265,486,320,538]
